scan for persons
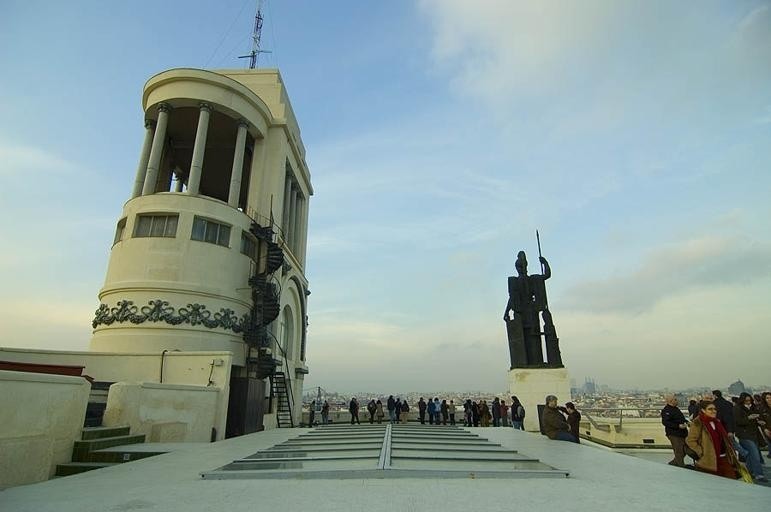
[504,249,552,368]
[309,399,316,428]
[349,395,582,443]
[660,388,771,485]
[320,400,329,426]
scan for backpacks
[517,406,525,418]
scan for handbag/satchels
[489,412,492,419]
[466,409,471,417]
[683,444,699,460]
[733,462,753,485]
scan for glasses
[708,407,717,410]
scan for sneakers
[757,477,769,483]
